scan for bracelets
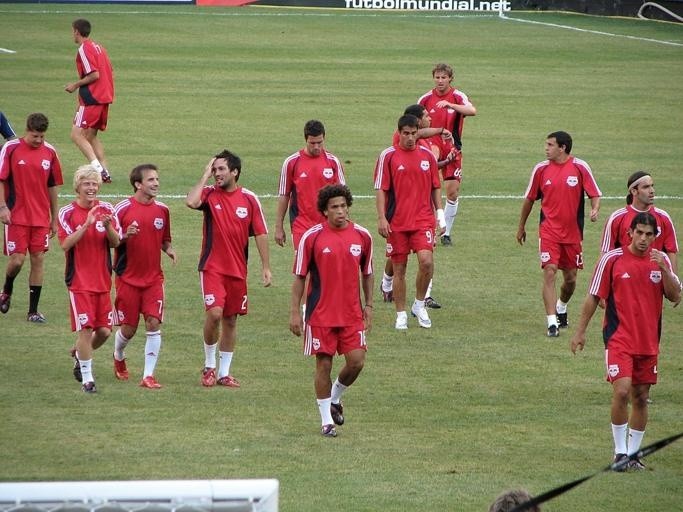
[439,127,444,135]
[436,209,444,219]
[0,206,6,210]
[364,304,373,308]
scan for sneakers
[0,289,12,313]
[394,313,408,330]
[25,312,47,324]
[329,400,345,426]
[546,312,569,338]
[379,280,393,303]
[609,452,646,473]
[70,347,82,383]
[409,305,433,330]
[215,375,241,387]
[423,296,441,310]
[200,365,215,387]
[82,383,98,393]
[100,168,112,184]
[440,233,450,246]
[111,351,129,381]
[320,423,337,437]
[138,375,161,389]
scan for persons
[594,169,680,406]
[0,104,16,145]
[489,487,541,511]
[514,130,603,339]
[378,103,463,310]
[372,113,449,332]
[184,150,273,390]
[286,182,375,439]
[108,164,179,391]
[273,119,347,327]
[0,113,65,327]
[415,63,477,248]
[569,211,682,474]
[56,163,123,396]
[63,18,116,184]
